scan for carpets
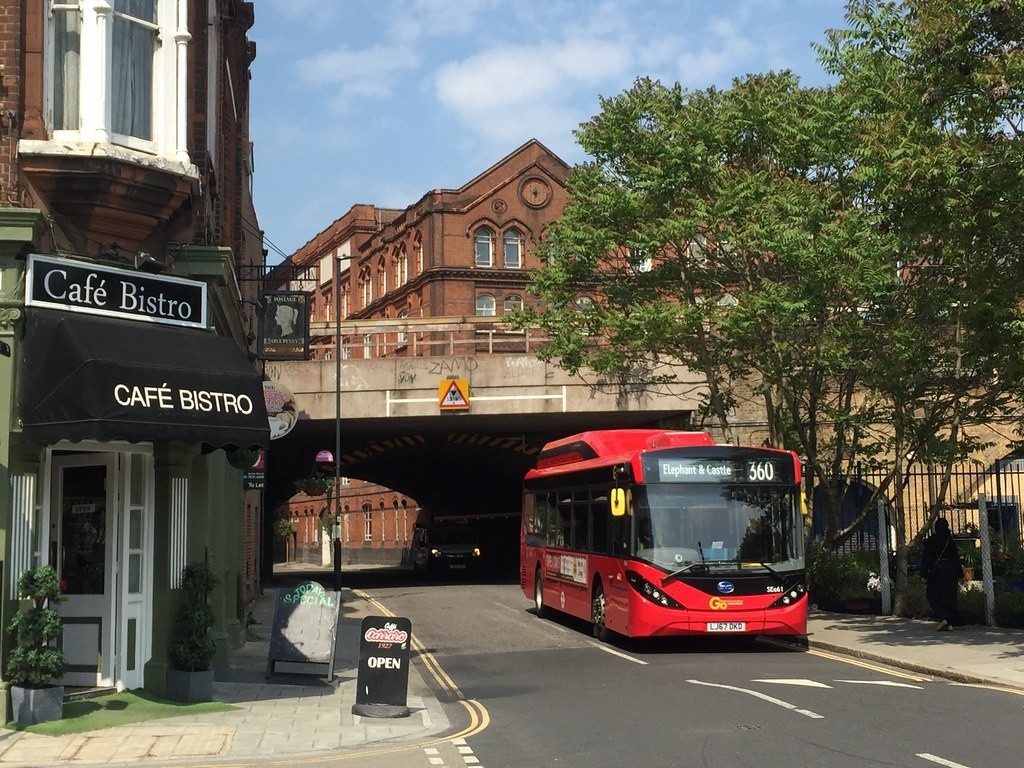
[0,686,243,737]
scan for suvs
[414,523,482,581]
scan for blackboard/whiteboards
[269,581,341,664]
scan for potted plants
[165,562,223,701]
[224,447,260,469]
[293,478,333,496]
[833,560,873,614]
[5,563,68,725]
[959,545,978,580]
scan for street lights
[334,254,362,592]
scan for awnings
[20,306,271,454]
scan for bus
[518,428,809,644]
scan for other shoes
[937,619,953,631]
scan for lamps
[110,242,164,275]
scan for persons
[920,518,964,632]
[639,518,656,549]
